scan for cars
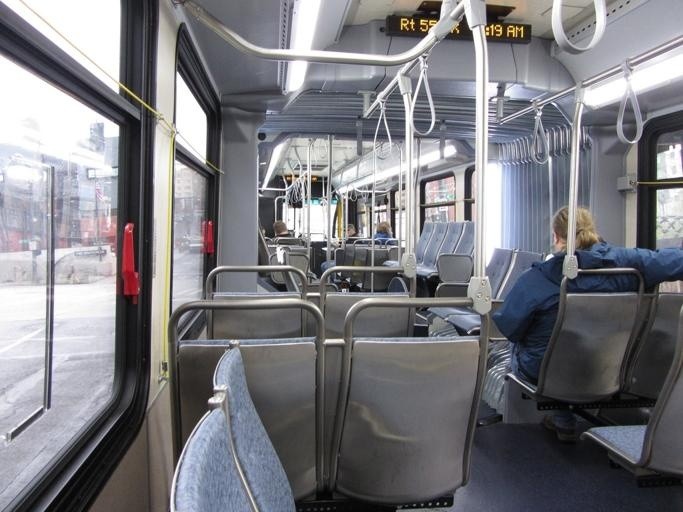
[187,238,203,252]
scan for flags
[96,183,105,202]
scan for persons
[258,220,309,292]
[322,222,365,277]
[490,205,682,444]
[362,221,399,294]
